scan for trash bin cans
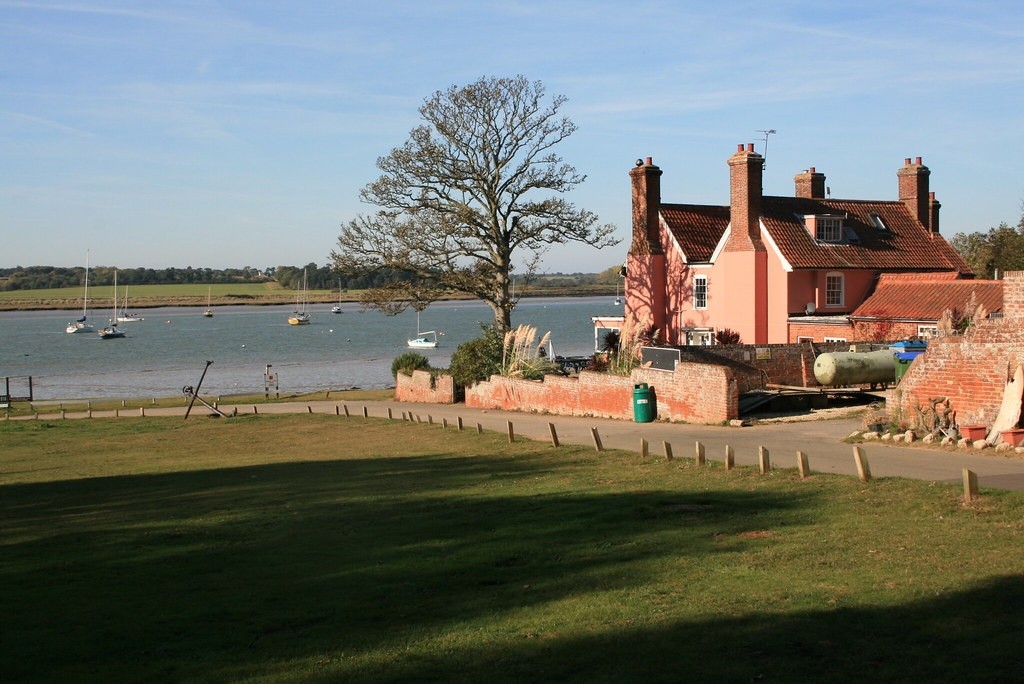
[888,338,928,388]
[632,382,654,423]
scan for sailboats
[331,277,343,314]
[98,270,127,339]
[407,291,440,348]
[66,248,95,333]
[614,282,622,306]
[203,285,213,317]
[287,268,311,325]
[117,285,145,322]
